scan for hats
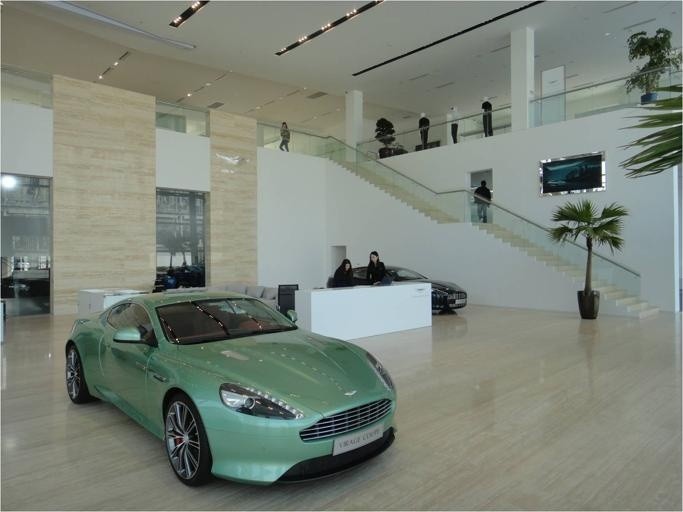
[325,265,467,314]
[154,264,203,290]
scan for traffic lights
[224,282,277,300]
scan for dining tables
[373,116,397,158]
[545,194,629,320]
[623,26,683,106]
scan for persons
[419,112,429,150]
[367,251,385,285]
[474,180,491,223]
[482,97,493,137]
[334,259,353,287]
[279,122,290,152]
[448,107,459,144]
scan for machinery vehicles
[63,288,397,488]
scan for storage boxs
[277,284,300,317]
